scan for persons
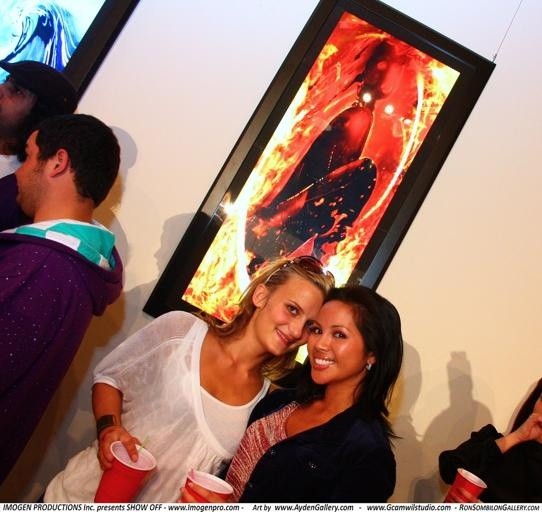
[36,255,335,503]
[439,377,542,503]
[176,286,403,503]
[0,60,78,231]
[0,114,122,485]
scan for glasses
[264,255,336,286]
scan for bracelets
[96,414,117,440]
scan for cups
[443,467,488,503]
[178,468,234,502]
[91,438,158,502]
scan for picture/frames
[143,1,496,389]
[0,0,139,163]
